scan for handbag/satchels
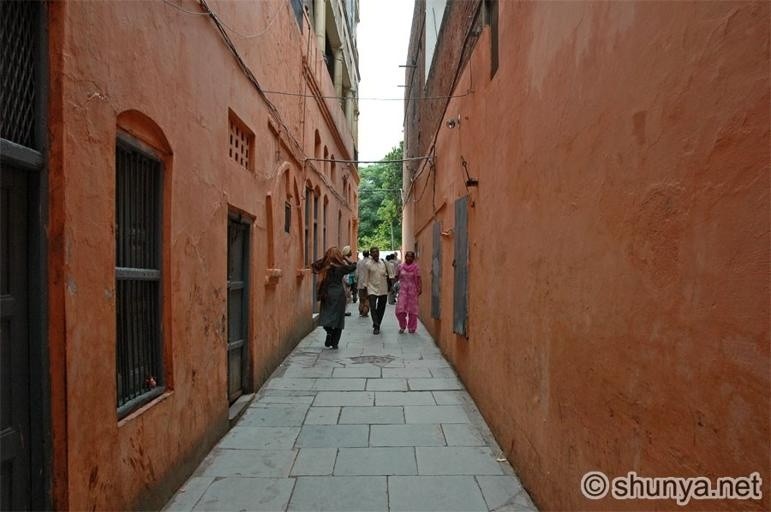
[316,278,324,301]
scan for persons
[318,246,423,350]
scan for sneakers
[325,334,339,349]
[399,327,415,334]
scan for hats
[342,245,352,258]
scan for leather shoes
[373,326,380,335]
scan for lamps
[446,114,463,132]
